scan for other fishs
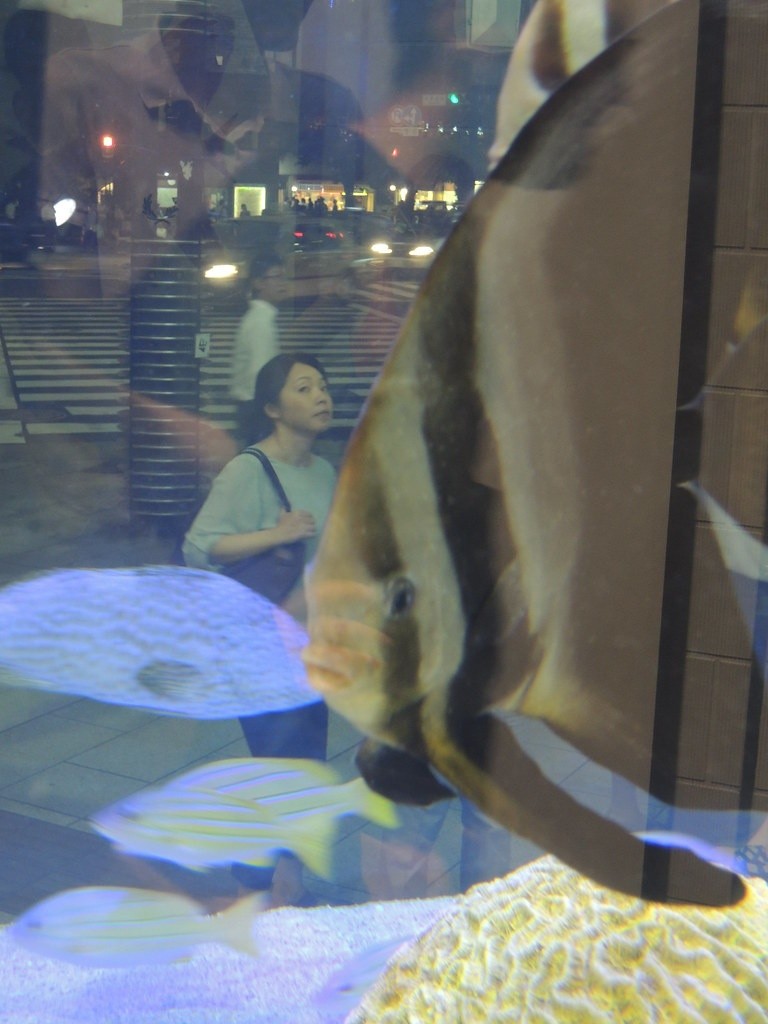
[1,2,768,1023]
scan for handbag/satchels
[210,446,304,652]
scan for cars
[294,222,346,248]
[0,214,99,262]
[347,222,437,279]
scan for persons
[181,353,337,906]
[229,254,287,444]
[240,205,250,215]
[98,199,131,237]
[283,197,337,211]
[215,200,226,217]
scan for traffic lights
[448,92,461,119]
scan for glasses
[261,272,286,281]
